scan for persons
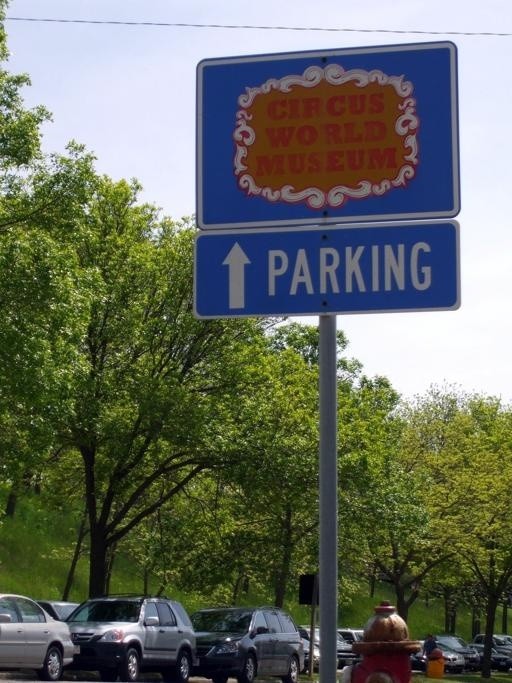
[422,633,437,659]
